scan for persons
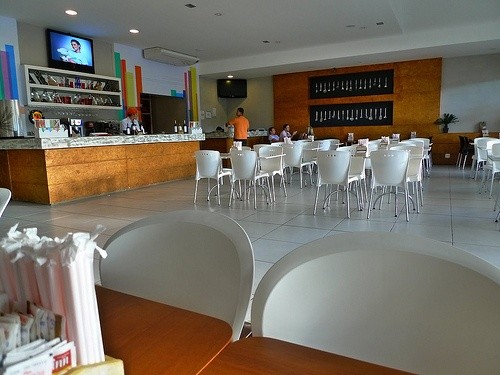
[279,124,297,141]
[61,39,88,65]
[121,107,146,135]
[227,108,249,146]
[268,127,283,144]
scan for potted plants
[434,113,459,133]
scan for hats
[127,107,138,115]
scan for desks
[91,285,232,375]
[196,337,417,375]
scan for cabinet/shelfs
[19,65,123,111]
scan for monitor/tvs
[217,79,247,98]
[45,28,95,74]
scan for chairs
[98,136,500,375]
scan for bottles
[174,119,178,133]
[61,75,111,91]
[178,123,183,133]
[66,118,72,136]
[183,119,188,134]
